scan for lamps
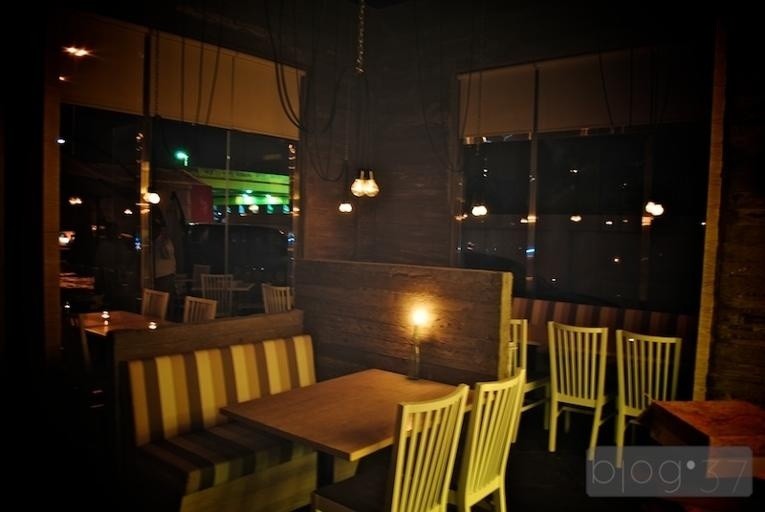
[350,0,381,198]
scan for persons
[137,204,176,301]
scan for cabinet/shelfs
[650,399,765,511]
